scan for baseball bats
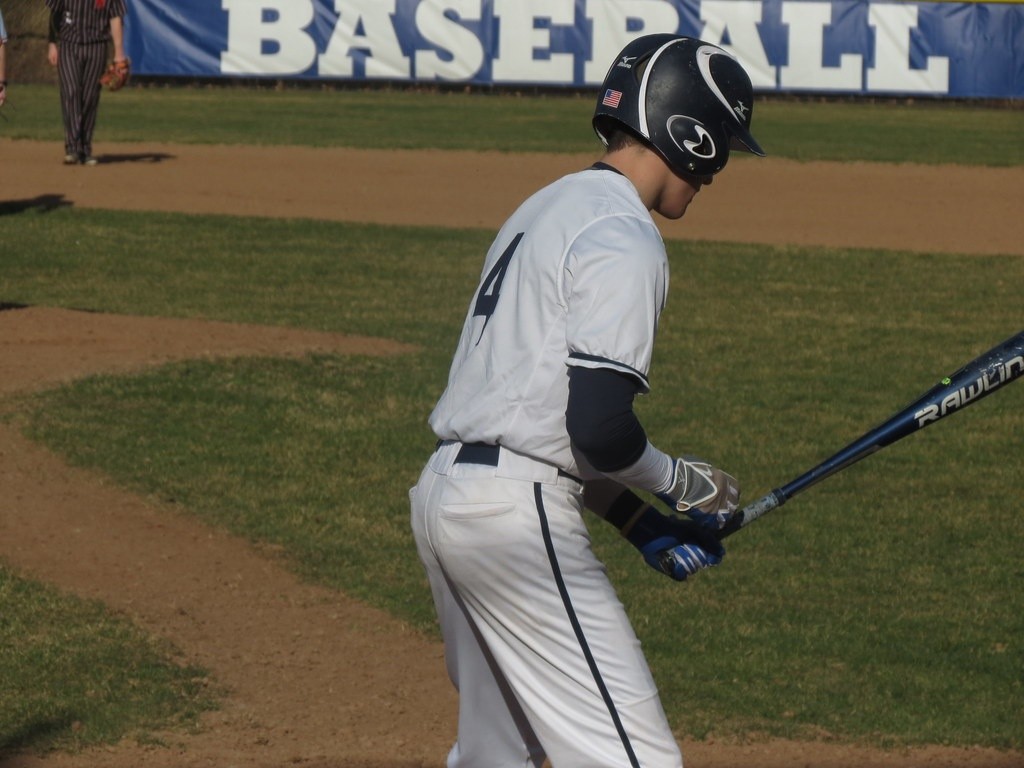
[654,329,1024,577]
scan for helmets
[592,31,768,178]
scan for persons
[45,0,132,166]
[407,33,767,768]
[0,9,8,106]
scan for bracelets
[0,81,7,86]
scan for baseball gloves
[99,55,132,93]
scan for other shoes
[63,153,99,168]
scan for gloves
[622,506,726,583]
[654,459,741,533]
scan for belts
[432,437,501,465]
